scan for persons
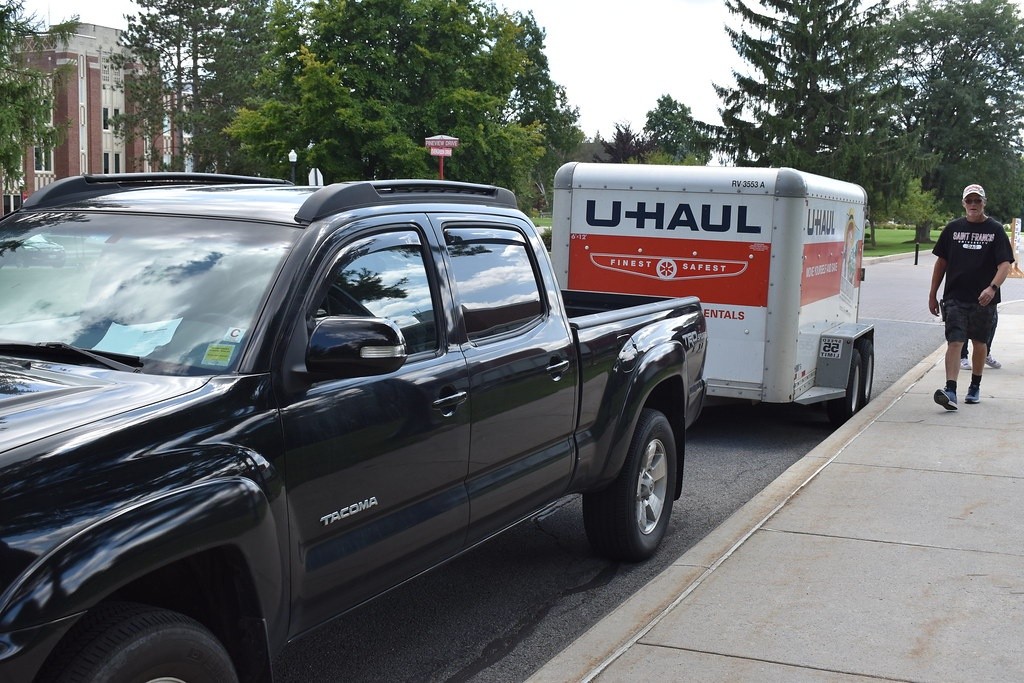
[929,184,1015,410]
[960,306,1001,370]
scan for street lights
[287,149,297,183]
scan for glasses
[964,198,983,204]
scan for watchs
[989,283,999,291]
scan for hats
[963,183,985,200]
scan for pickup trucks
[0,164,708,683]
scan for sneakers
[964,383,981,403]
[960,358,972,370]
[933,388,958,411]
[986,353,1001,368]
